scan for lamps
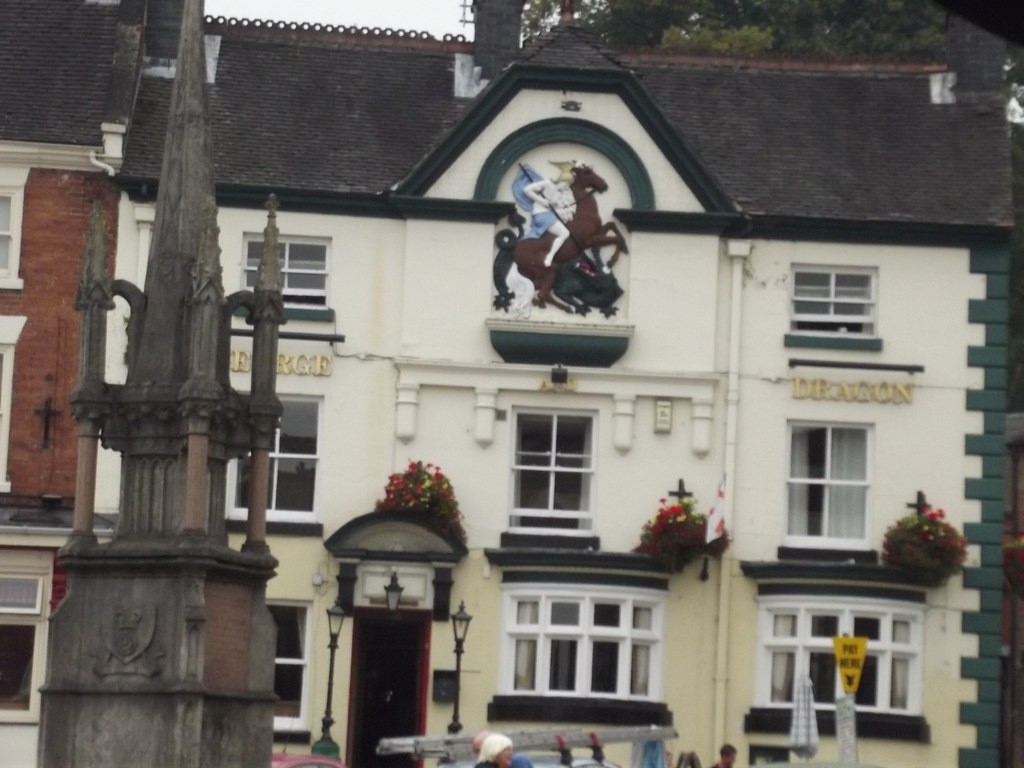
[314,560,330,589]
[382,564,404,613]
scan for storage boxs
[433,670,456,702]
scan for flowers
[376,459,461,526]
[639,495,710,571]
[882,508,968,571]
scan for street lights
[309,600,346,763]
[450,604,474,738]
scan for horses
[512,165,629,312]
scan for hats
[473,734,512,763]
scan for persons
[522,170,574,266]
[473,731,533,768]
[666,744,736,768]
